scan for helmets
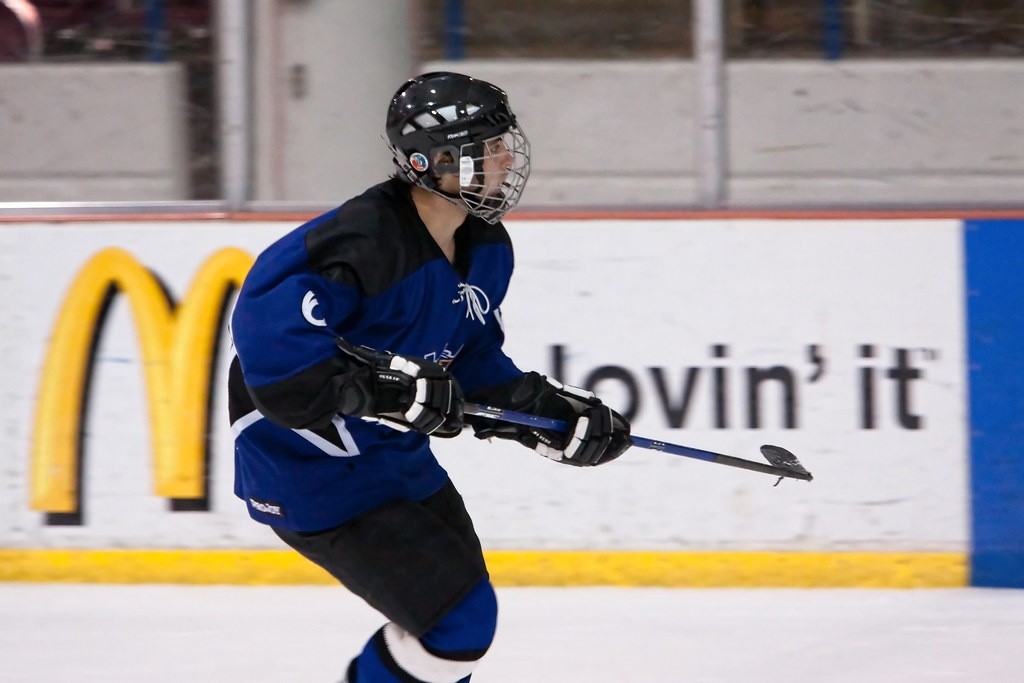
[387,71,531,223]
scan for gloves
[473,368,632,467]
[331,334,465,438]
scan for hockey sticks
[338,381,815,483]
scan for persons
[228,72,633,683]
